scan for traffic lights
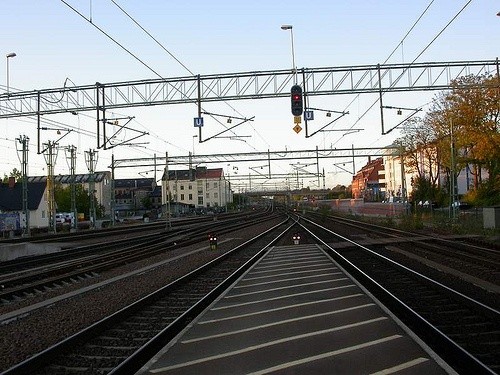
[291,84,303,116]
